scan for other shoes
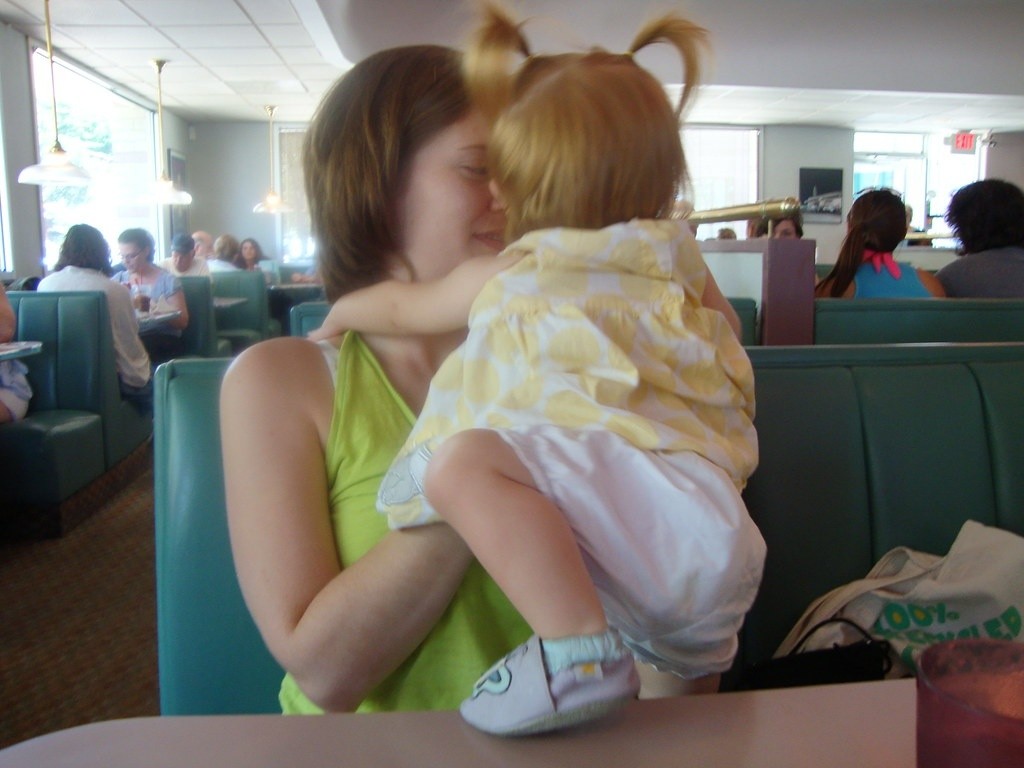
[459,636,640,735]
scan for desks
[215,297,248,308]
[0,341,43,362]
[271,282,320,291]
[138,310,181,331]
[0,664,1024,768]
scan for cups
[912,636,1024,768]
[132,284,151,315]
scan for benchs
[1,235,1024,714]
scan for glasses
[121,248,145,260]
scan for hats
[170,233,194,251]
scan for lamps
[17,1,91,185]
[943,130,984,154]
[252,104,293,214]
[128,60,191,203]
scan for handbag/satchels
[736,617,914,688]
[770,519,1024,664]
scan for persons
[0,225,280,402]
[894,179,1024,298]
[818,190,943,299]
[717,214,822,287]
[676,200,698,239]
[218,46,532,714]
[0,279,32,425]
[305,10,762,738]
[291,262,323,284]
